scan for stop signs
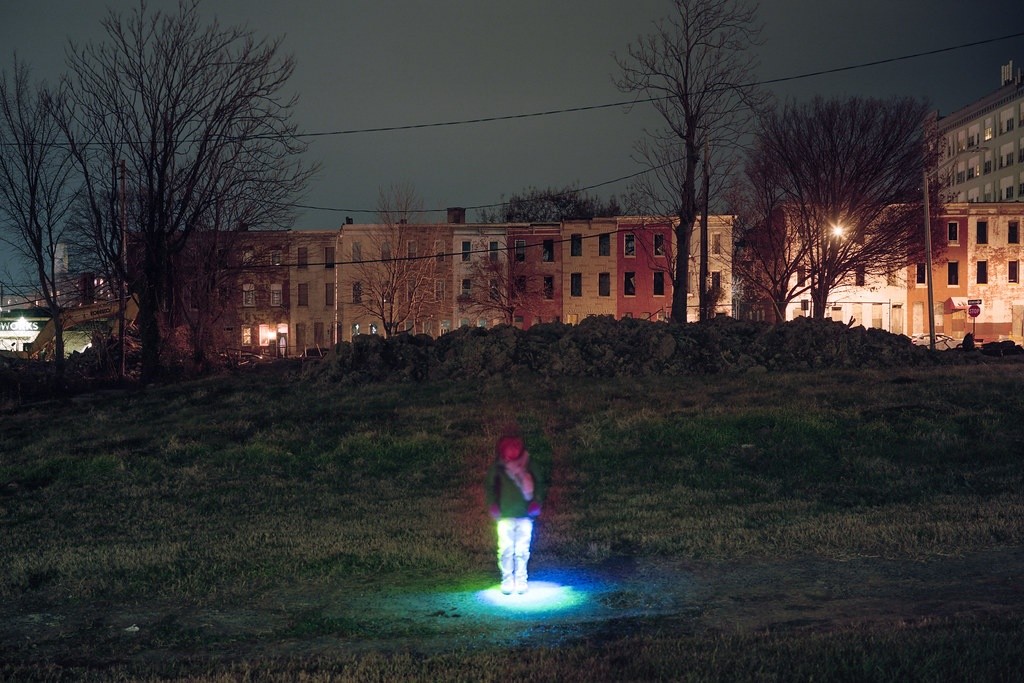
[969,305,980,317]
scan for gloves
[528,501,540,516]
[487,504,501,518]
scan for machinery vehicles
[0,293,139,371]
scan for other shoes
[501,580,513,594]
[515,580,528,594]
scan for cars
[913,333,965,350]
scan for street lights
[921,144,991,351]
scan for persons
[486,433,545,595]
